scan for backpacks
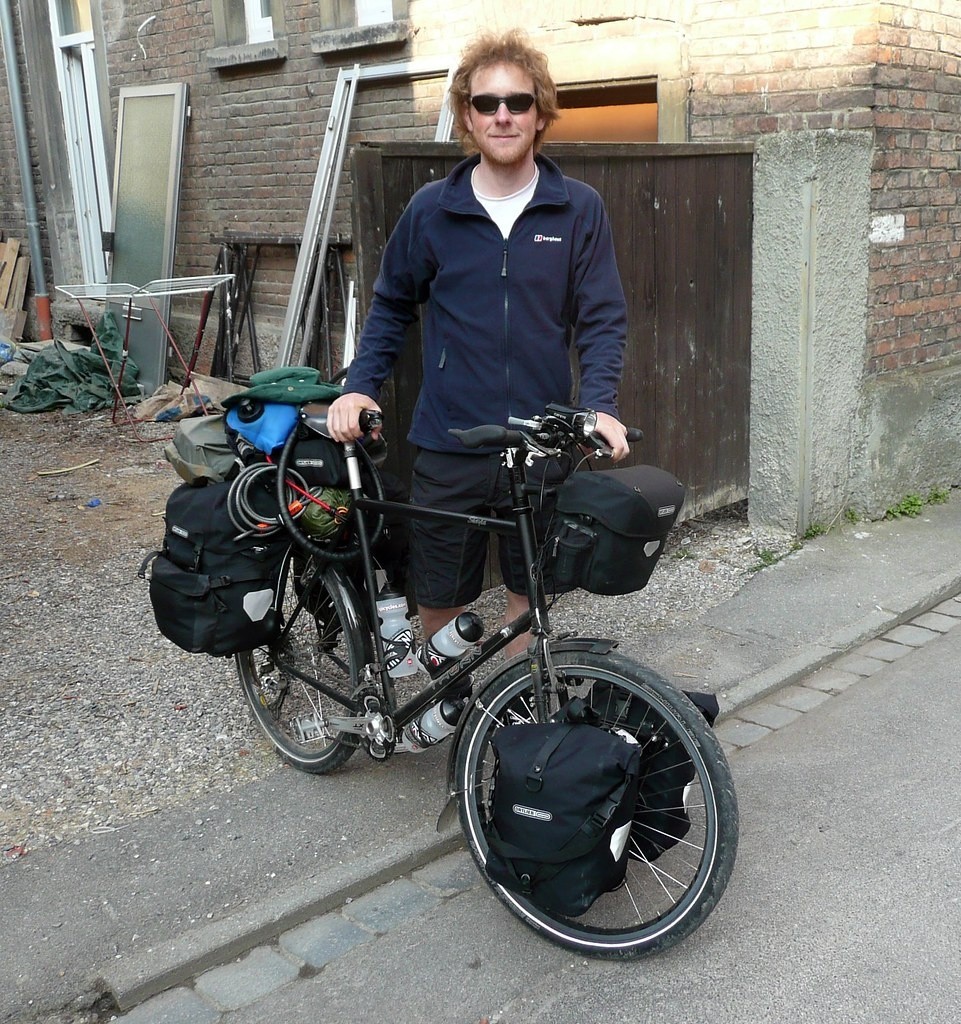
[138,480,281,659]
[585,674,718,856]
[485,720,639,917]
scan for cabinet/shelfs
[53,274,236,442]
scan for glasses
[466,93,535,113]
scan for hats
[221,365,344,409]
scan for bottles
[402,696,462,753]
[375,583,418,677]
[415,611,484,673]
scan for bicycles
[234,403,739,962]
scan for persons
[327,34,631,757]
